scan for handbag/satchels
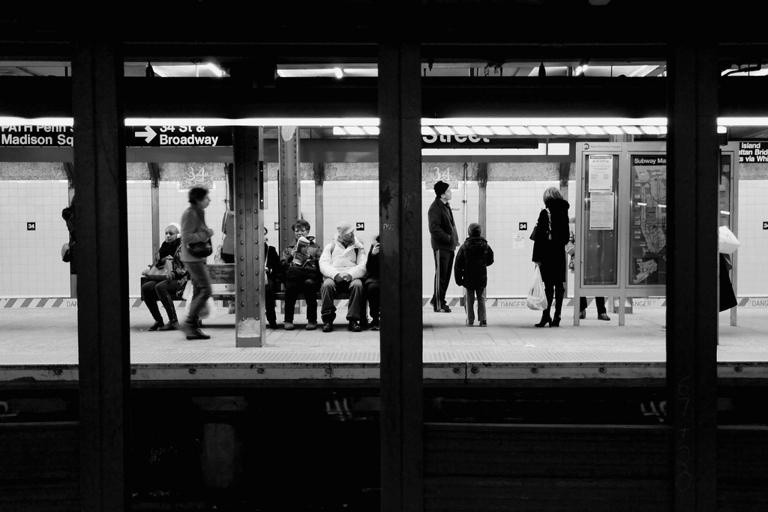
[530,225,553,242]
[146,257,173,280]
[719,225,740,255]
[214,244,222,262]
[186,239,213,259]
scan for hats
[337,221,355,237]
[433,181,449,196]
[468,223,481,237]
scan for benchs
[207,262,348,300]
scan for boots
[535,289,553,328]
[552,288,566,327]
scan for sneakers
[285,321,294,330]
[431,300,451,312]
[579,311,586,319]
[186,329,210,339]
[306,321,317,330]
[598,313,610,320]
[322,321,332,332]
[369,319,381,330]
[479,320,487,327]
[347,321,361,332]
[147,319,165,331]
